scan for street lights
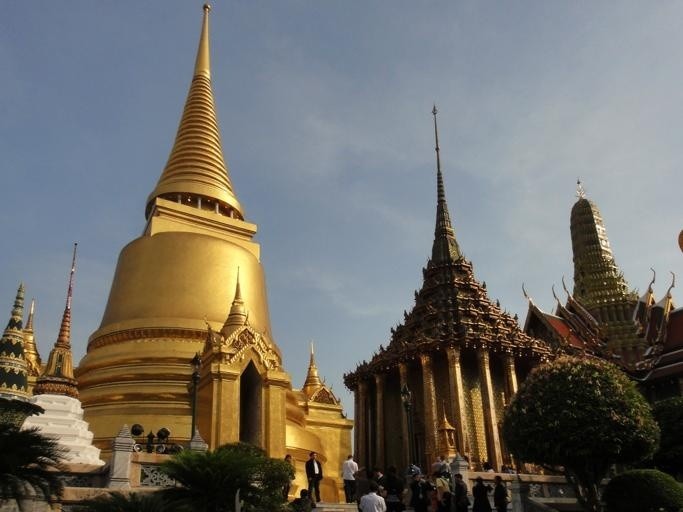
[400,384,413,473]
[191,350,203,436]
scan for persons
[290,489,316,512]
[285,454,292,462]
[358,455,507,512]
[342,455,358,503]
[306,452,322,502]
